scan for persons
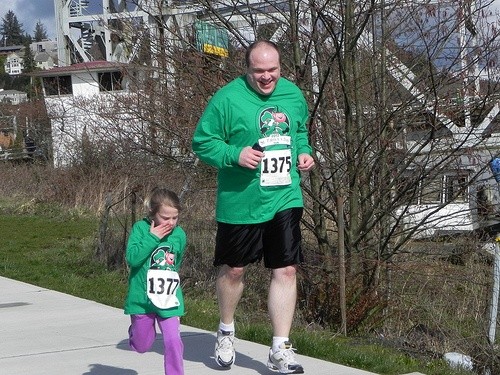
[124,186,186,375]
[192,40,314,374]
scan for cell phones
[252,143,264,152]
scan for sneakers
[214,328,236,367]
[266,341,305,375]
